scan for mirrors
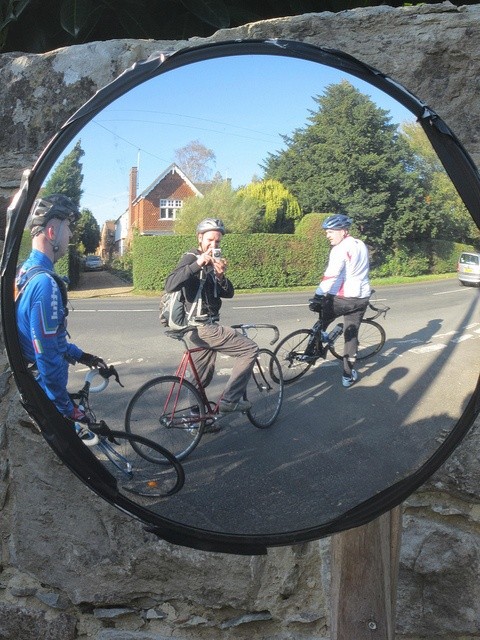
[1,36,480,556]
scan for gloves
[79,353,102,367]
[309,293,324,311]
[71,407,90,424]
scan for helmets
[197,218,225,235]
[322,214,352,230]
[29,194,82,230]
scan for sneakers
[191,421,220,436]
[342,369,357,387]
[219,400,252,414]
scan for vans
[456,252,479,288]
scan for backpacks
[160,252,208,339]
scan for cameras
[212,249,221,259]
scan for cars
[84,256,103,272]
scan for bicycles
[124,323,284,465]
[269,290,391,385]
[42,365,185,500]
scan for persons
[14,192,105,441]
[307,213,373,388]
[158,217,260,432]
